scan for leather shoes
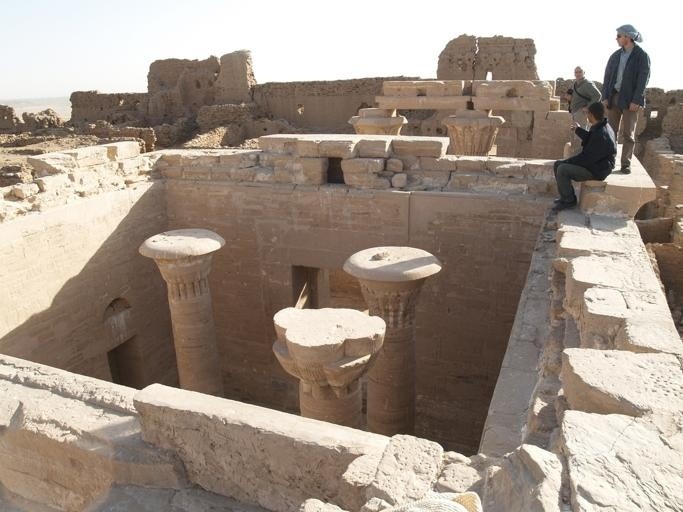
[552,196,576,211]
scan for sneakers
[621,165,631,174]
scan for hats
[616,25,643,42]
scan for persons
[561,66,602,160]
[551,102,617,211]
[600,24,652,175]
